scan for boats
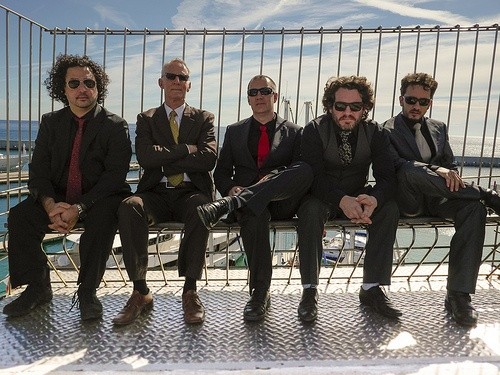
[5,230,246,288]
[271,228,408,270]
[129,161,144,170]
[0,153,33,172]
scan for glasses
[247,87,274,96]
[66,79,97,89]
[334,101,365,112]
[162,72,190,81]
[402,96,430,106]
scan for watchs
[75,202,85,219]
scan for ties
[257,125,270,179]
[338,130,352,168]
[167,111,184,186]
[66,117,86,202]
[413,122,432,164]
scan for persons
[197,76,314,322]
[112,59,218,326]
[297,75,403,320]
[3,53,132,323]
[380,72,500,328]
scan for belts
[157,181,192,189]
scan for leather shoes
[3,284,53,317]
[444,296,479,326]
[297,288,319,322]
[67,284,103,320]
[196,196,230,231]
[484,186,500,216]
[359,285,403,318]
[182,289,205,324]
[244,288,271,321]
[111,288,154,325]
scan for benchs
[3,211,500,286]
